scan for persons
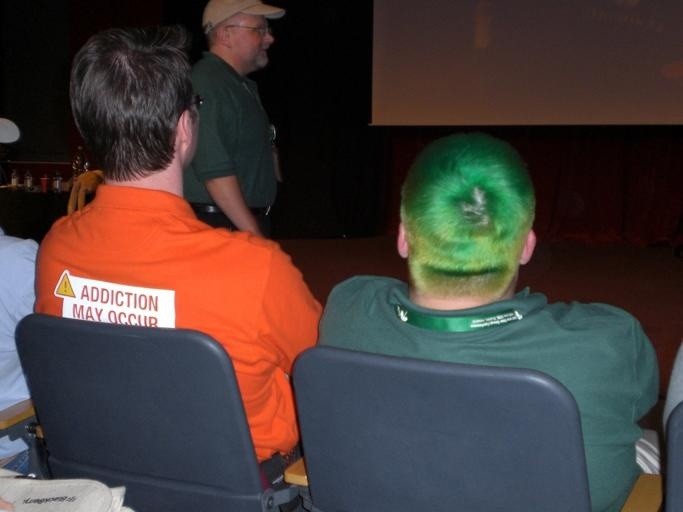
[317,132,659,511]
[182,2,286,242]
[34,22,323,481]
[2,118,40,478]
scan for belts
[188,202,273,215]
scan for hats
[201,1,285,36]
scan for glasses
[186,93,205,106]
[225,24,271,35]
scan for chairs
[282,343,593,512]
[14,313,306,511]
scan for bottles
[72,145,88,182]
[11,168,63,192]
